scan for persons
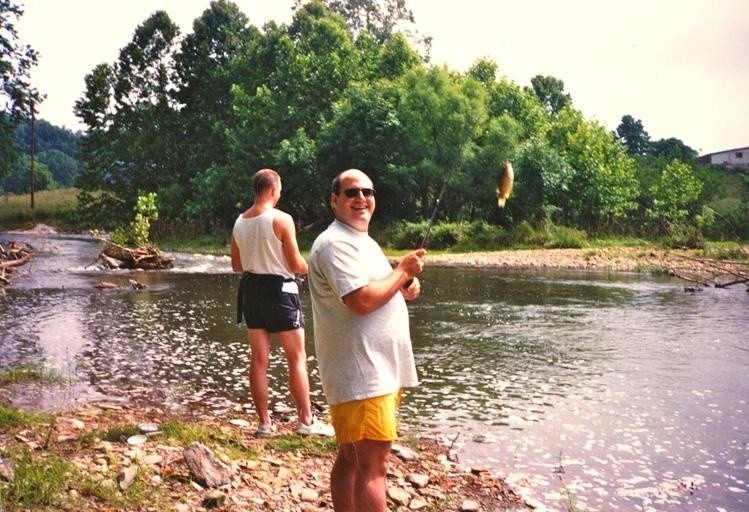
[230,168,335,437]
[308,169,426,512]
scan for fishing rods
[399,70,511,292]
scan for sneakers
[257,421,276,437]
[296,414,335,437]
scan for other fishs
[495,158,514,209]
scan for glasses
[344,188,376,198]
